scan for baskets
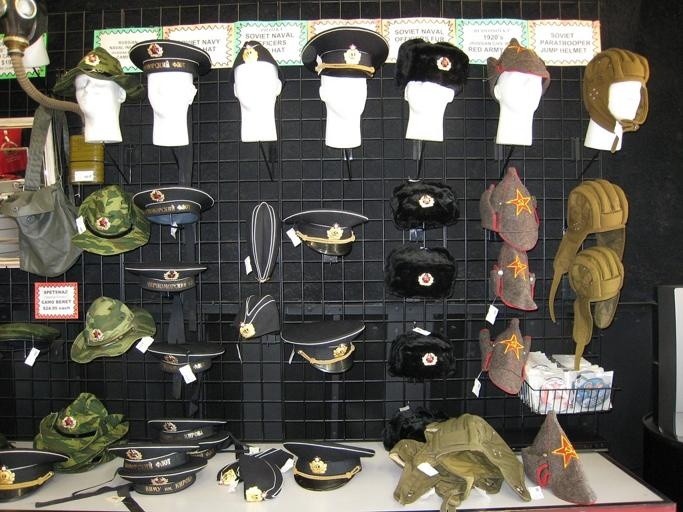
[520,379,612,414]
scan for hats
[300,27,390,80]
[280,320,366,373]
[582,48,650,133]
[51,46,146,104]
[485,242,538,311]
[412,413,531,502]
[71,184,151,256]
[479,318,532,395]
[383,243,457,298]
[1,321,62,350]
[486,38,553,102]
[147,343,225,374]
[568,246,625,371]
[480,167,540,251]
[395,39,472,96]
[388,332,456,380]
[70,296,157,364]
[283,209,369,256]
[391,182,458,231]
[130,38,211,77]
[124,265,208,292]
[33,393,230,495]
[132,186,215,224]
[217,448,294,502]
[521,410,598,505]
[388,439,504,512]
[235,294,281,339]
[282,442,375,491]
[549,178,629,323]
[382,405,449,451]
[0,448,70,503]
[244,201,281,284]
[227,41,285,96]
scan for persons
[301,27,390,149]
[233,41,282,142]
[130,39,210,147]
[397,38,469,143]
[55,47,145,143]
[488,38,550,147]
[583,48,650,153]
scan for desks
[2,445,678,511]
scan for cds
[539,377,574,413]
[575,379,612,409]
[532,364,552,372]
[572,371,598,394]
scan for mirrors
[0,116,57,267]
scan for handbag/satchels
[2,96,85,279]
